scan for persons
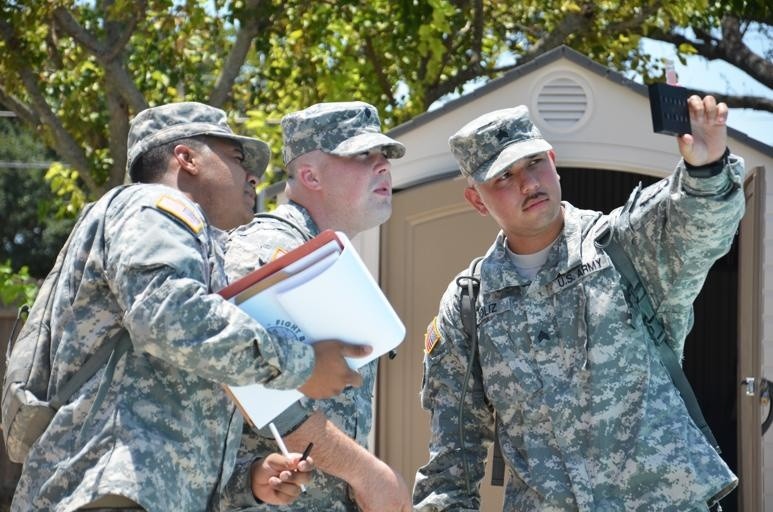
[209,101,413,511]
[407,72,746,511]
[0,101,374,511]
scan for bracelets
[683,151,735,178]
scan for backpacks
[0,202,93,464]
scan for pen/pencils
[269,422,307,496]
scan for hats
[447,105,552,188]
[141,102,270,180]
[280,100,406,160]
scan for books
[210,232,411,446]
